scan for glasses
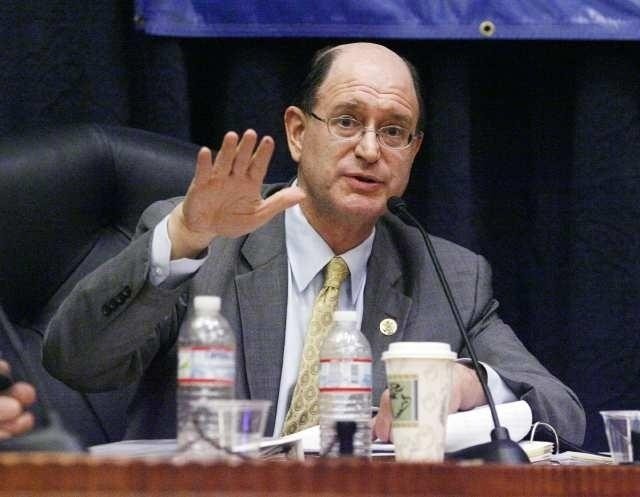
[300,104,425,153]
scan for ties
[280,256,350,460]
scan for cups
[219,398,273,455]
[600,410,640,463]
[381,340,457,466]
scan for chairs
[0,122,218,446]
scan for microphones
[386,195,532,465]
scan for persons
[1,360,38,440]
[40,42,588,454]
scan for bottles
[319,311,372,460]
[177,294,237,463]
